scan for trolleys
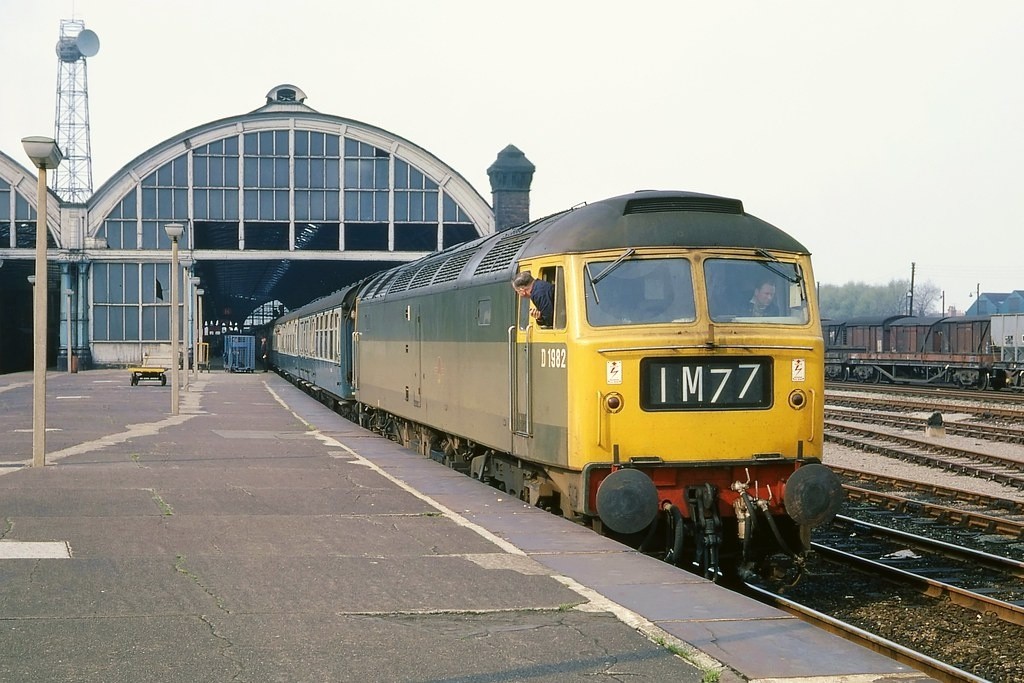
[127,347,168,387]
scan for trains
[242,189,844,602]
[821,313,1024,393]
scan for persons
[511,272,555,326]
[746,279,780,316]
[260,336,269,373]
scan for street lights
[164,222,186,416]
[19,135,64,466]
[180,257,195,393]
